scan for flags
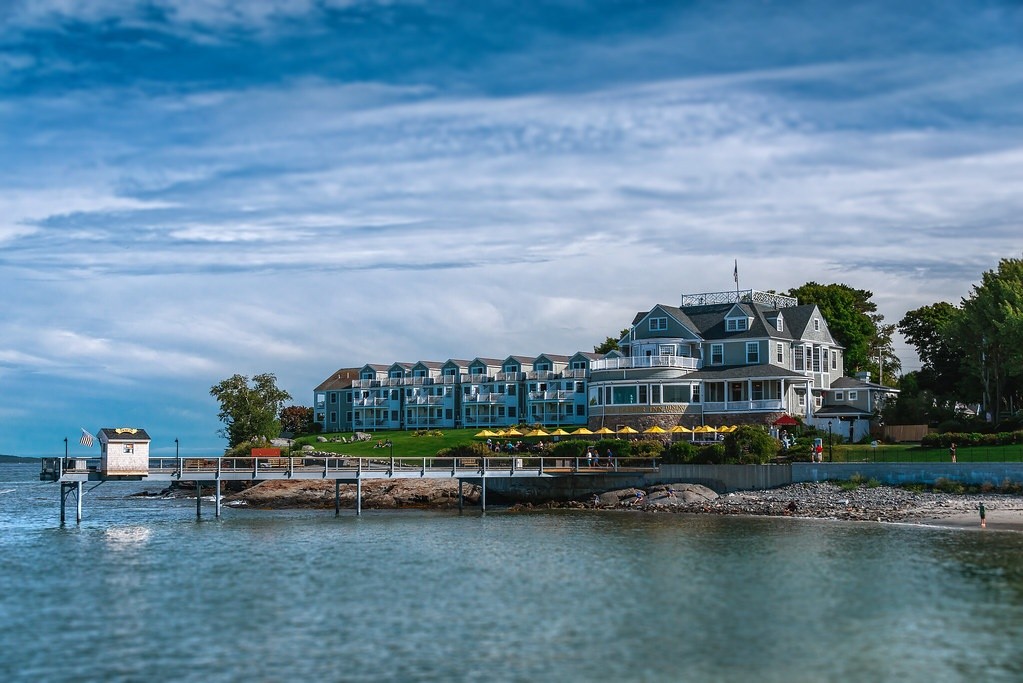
[79,431,94,448]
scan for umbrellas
[725,424,739,433]
[524,429,548,444]
[474,429,499,440]
[643,426,668,440]
[694,426,702,440]
[494,429,505,442]
[694,425,716,441]
[716,425,728,432]
[772,414,800,430]
[594,427,616,439]
[569,428,595,441]
[548,429,570,441]
[616,426,639,441]
[502,428,524,440]
[668,426,692,441]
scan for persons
[372,440,383,449]
[607,448,614,468]
[979,501,986,527]
[782,430,788,448]
[816,445,824,464]
[261,435,267,443]
[486,439,562,455]
[593,450,601,467]
[790,433,795,446]
[810,444,816,463]
[251,435,258,443]
[382,438,391,448]
[611,433,725,447]
[586,448,593,467]
[950,442,957,461]
